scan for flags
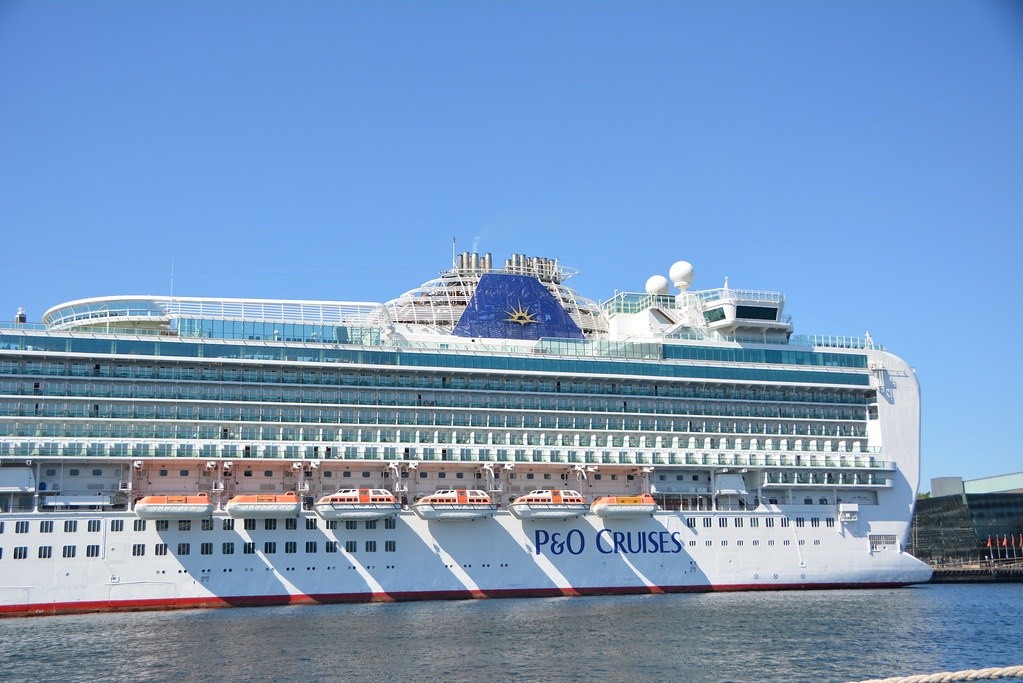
[1011,536,1014,545]
[987,536,990,547]
[996,537,999,547]
[1019,536,1022,547]
[1002,536,1007,547]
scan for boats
[590,494,658,517]
[316,488,401,522]
[135,493,214,520]
[505,490,592,519]
[411,490,498,525]
[226,492,301,519]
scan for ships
[0,240,935,617]
